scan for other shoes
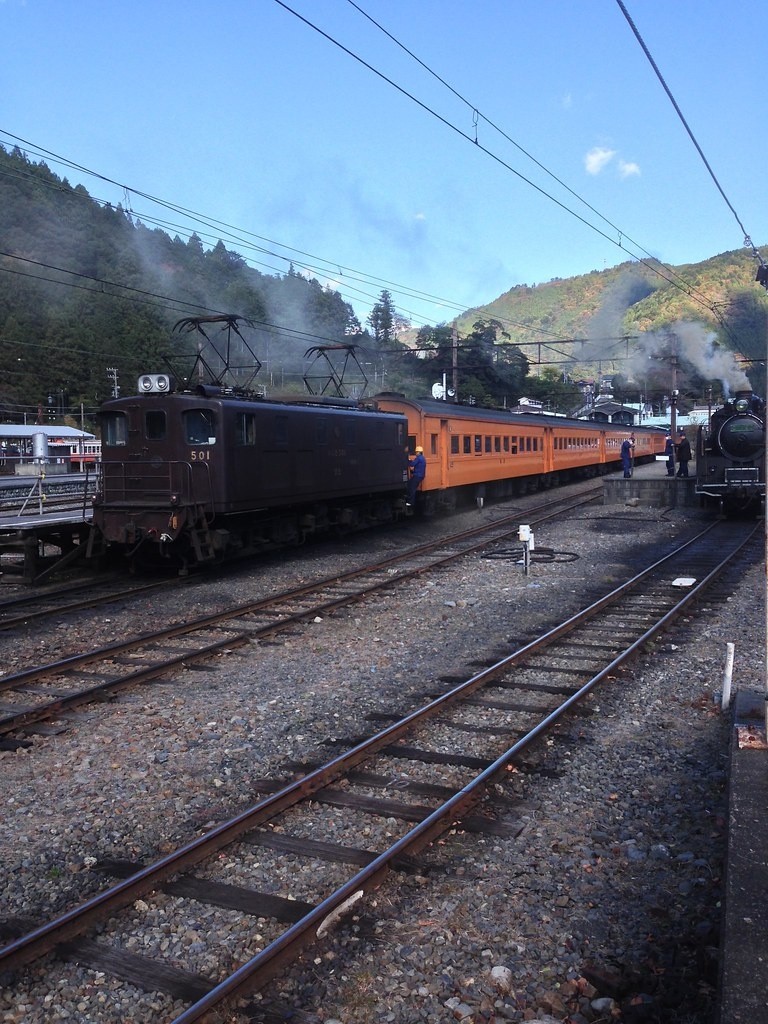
[676,473,681,477]
[683,474,688,478]
[625,475,630,478]
[406,503,413,506]
[665,473,674,477]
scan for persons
[405,446,427,507]
[672,433,692,476]
[620,438,635,478]
[665,433,674,477]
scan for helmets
[680,431,686,436]
[666,433,671,436]
[415,446,423,452]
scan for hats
[629,437,635,443]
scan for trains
[86,313,683,577]
[694,389,766,518]
